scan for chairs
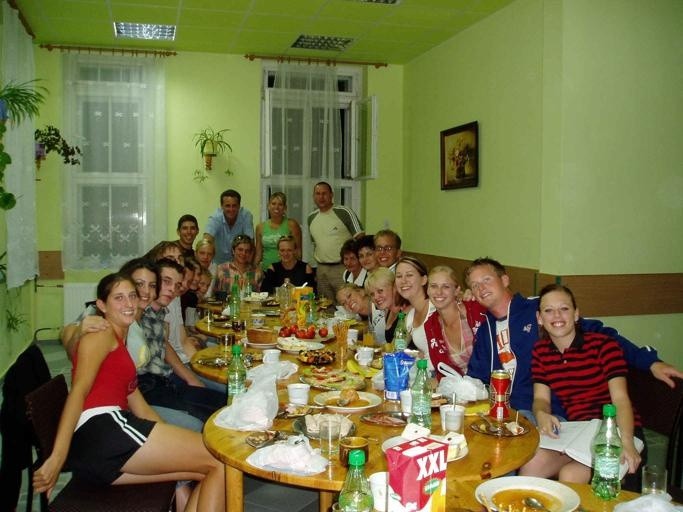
[630,370,683,505]
[22,374,179,507]
[0,343,53,507]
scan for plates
[379,433,471,464]
[289,332,335,343]
[244,429,303,450]
[311,389,384,415]
[473,474,582,511]
[240,337,280,349]
[361,411,413,428]
[251,306,280,316]
[195,351,256,369]
[468,418,532,439]
[295,357,337,365]
[213,314,231,321]
[297,369,368,391]
[242,296,276,302]
[290,412,359,442]
[275,340,328,355]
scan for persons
[334,283,374,321]
[364,267,412,350]
[195,232,216,297]
[461,256,683,477]
[147,241,231,393]
[258,235,317,299]
[357,235,377,271]
[211,234,265,296]
[138,258,228,393]
[373,228,402,273]
[183,240,228,324]
[517,284,646,484]
[423,264,487,384]
[253,191,302,272]
[202,188,255,265]
[31,274,227,511]
[306,180,364,301]
[141,214,198,259]
[394,256,475,357]
[338,237,368,288]
[59,257,205,432]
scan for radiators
[63,283,97,327]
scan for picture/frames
[438,118,480,191]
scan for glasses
[234,234,251,241]
[374,247,397,253]
[279,235,294,239]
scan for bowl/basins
[291,286,313,301]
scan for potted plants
[34,127,83,167]
[0,77,52,332]
[196,126,234,156]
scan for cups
[286,383,310,406]
[362,325,377,346]
[443,411,465,433]
[332,318,351,347]
[640,463,668,498]
[231,319,247,333]
[260,348,282,365]
[218,333,237,355]
[319,421,341,462]
[318,294,328,309]
[354,346,375,367]
[203,309,215,323]
[338,436,370,469]
[347,328,359,347]
[398,388,413,418]
[438,404,468,434]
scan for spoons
[477,411,500,433]
[525,496,552,511]
[308,396,351,412]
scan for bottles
[228,346,246,404]
[233,275,240,294]
[244,272,252,297]
[306,293,316,327]
[591,405,622,499]
[411,360,432,430]
[490,370,511,419]
[281,278,297,326]
[340,450,373,512]
[230,287,240,321]
[394,314,407,351]
